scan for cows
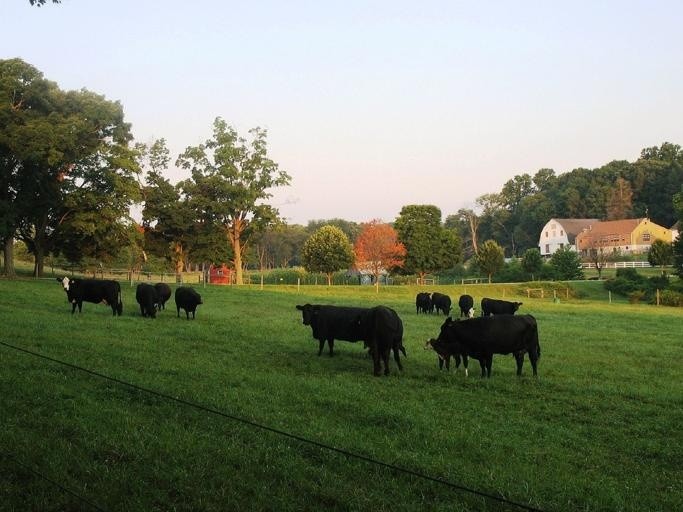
[296,303,407,358]
[136,282,172,319]
[415,291,542,379]
[56,276,123,317]
[357,305,404,377]
[174,286,203,320]
